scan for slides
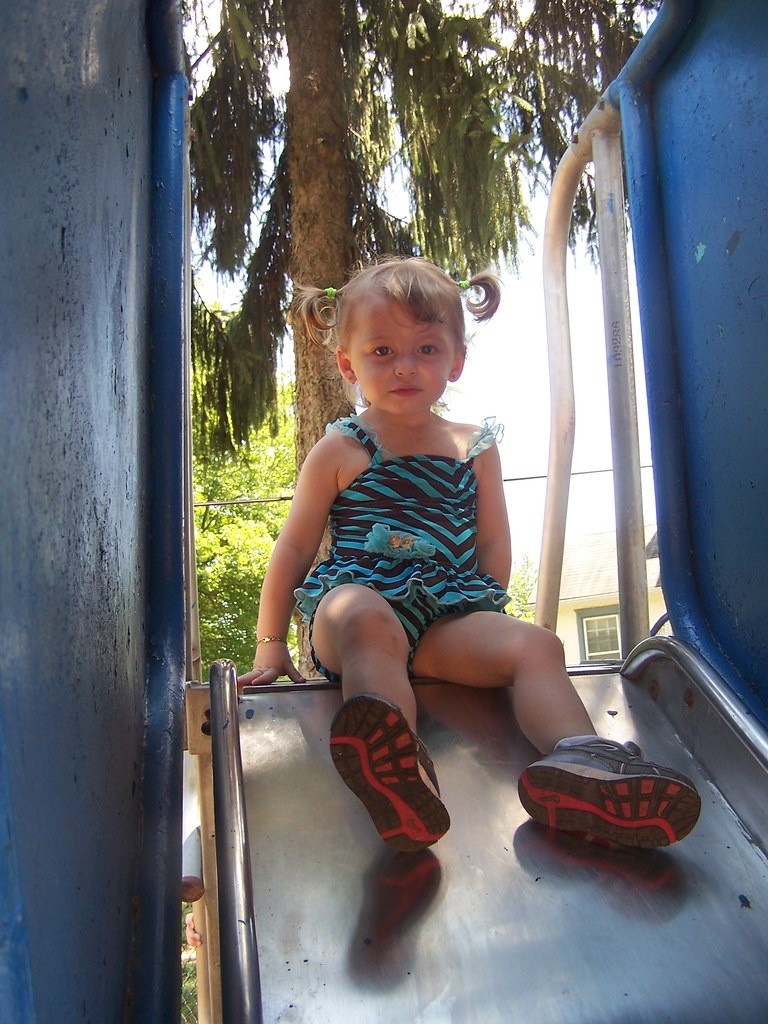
[201,627,768,1017]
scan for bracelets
[256,637,287,643]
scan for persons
[237,256,701,847]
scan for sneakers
[518,739,701,849]
[329,692,451,853]
